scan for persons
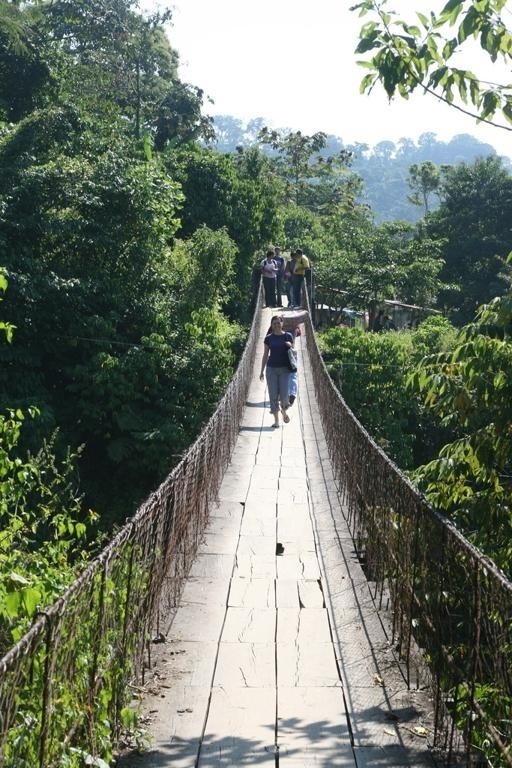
[291,249,309,309]
[270,319,301,408]
[260,250,277,307]
[271,247,285,308]
[258,315,298,428]
[283,251,296,307]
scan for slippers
[280,410,291,424]
[271,424,280,428]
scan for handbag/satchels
[284,332,298,373]
